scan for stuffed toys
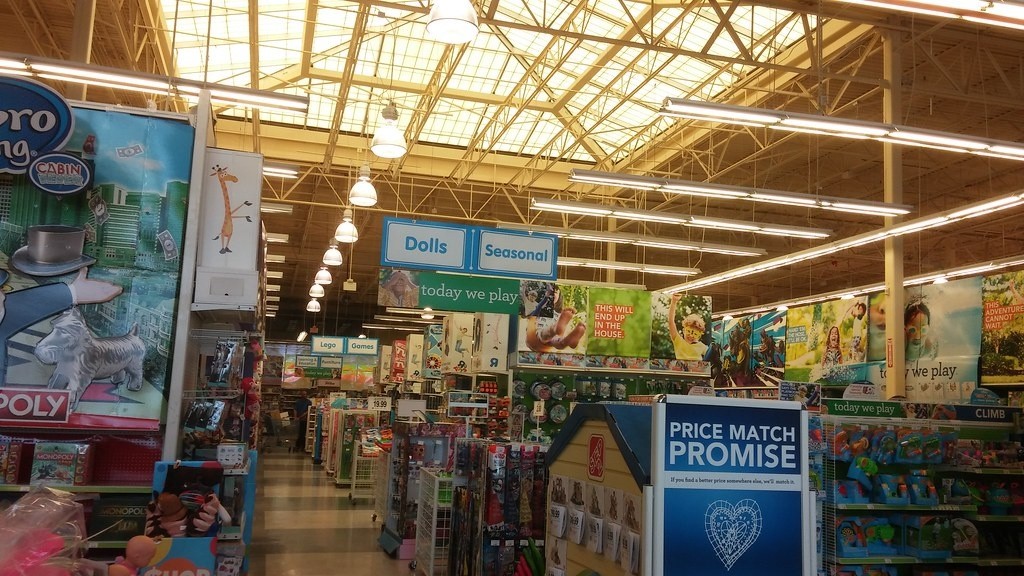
[143,465,232,538]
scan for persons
[526,285,586,353]
[522,281,553,316]
[794,385,820,406]
[821,300,868,370]
[904,302,932,361]
[294,391,312,452]
[411,345,421,368]
[108,535,156,576]
[669,294,709,362]
[454,319,473,357]
[378,271,419,309]
[475,319,481,352]
[1009,278,1024,304]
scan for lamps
[348,125,377,206]
[314,266,332,284]
[370,18,407,158]
[306,298,321,312]
[421,307,434,320]
[334,159,358,243]
[322,240,342,266]
[309,284,325,297]
[358,301,366,338]
[425,0,479,44]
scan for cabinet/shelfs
[233,358,1024,576]
